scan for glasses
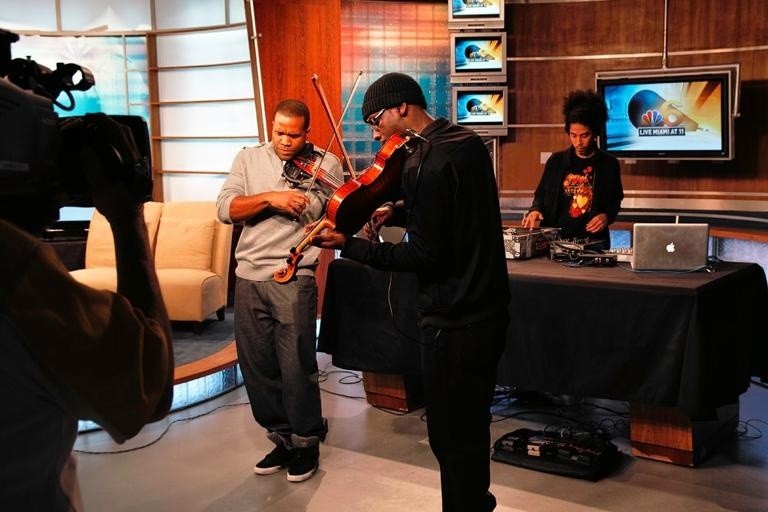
[369,108,385,125]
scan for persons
[302,72,510,511]
[215,98,345,484]
[0,111,176,512]
[521,88,624,250]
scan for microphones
[405,127,431,145]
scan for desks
[327,258,761,469]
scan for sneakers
[287,434,320,483]
[254,431,291,483]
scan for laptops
[630,222,711,272]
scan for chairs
[68,201,234,336]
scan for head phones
[550,241,585,267]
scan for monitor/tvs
[447,0,506,29]
[596,73,729,158]
[452,86,508,137]
[449,33,507,83]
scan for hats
[362,73,427,123]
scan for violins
[283,142,345,189]
[273,131,415,285]
